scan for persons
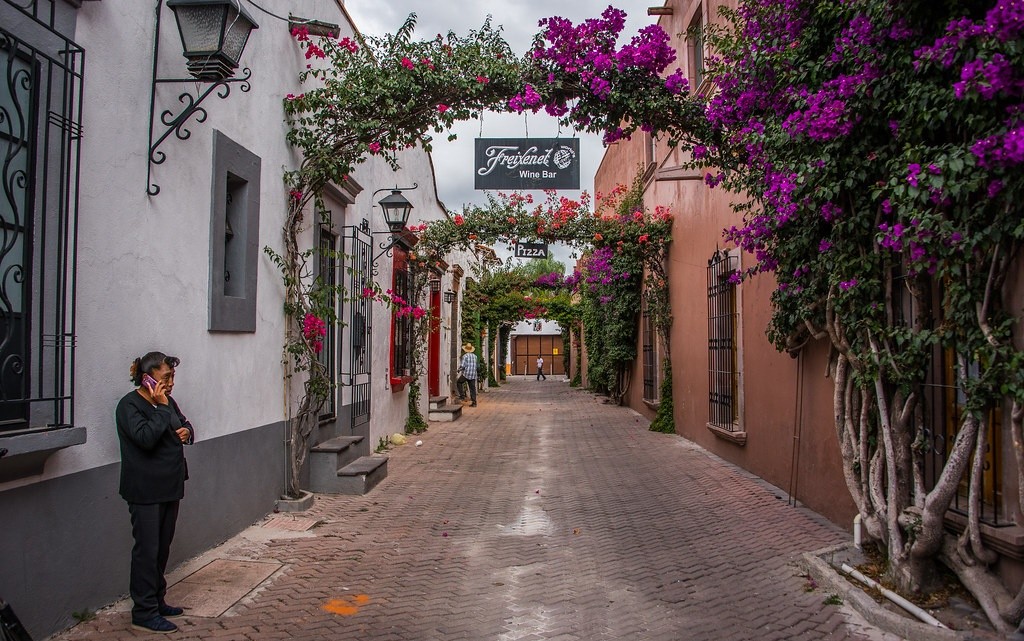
[457,343,478,406]
[116,352,195,633]
[535,354,546,382]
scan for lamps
[146,0,259,196]
[442,283,455,304]
[370,182,418,276]
[422,270,441,301]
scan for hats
[462,343,476,353]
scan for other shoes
[161,606,184,618]
[469,402,478,407]
[458,397,467,401]
[131,615,178,634]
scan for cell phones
[142,375,158,390]
